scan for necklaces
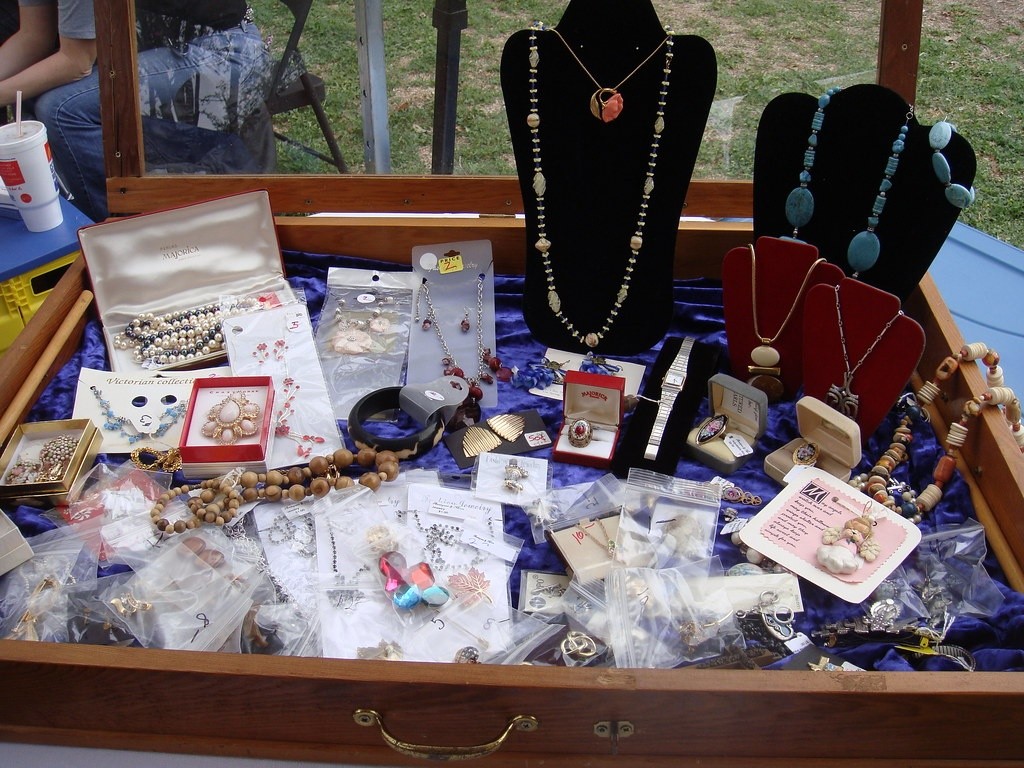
[4,234,1024,672]
[786,85,916,280]
[546,22,670,122]
[526,22,676,348]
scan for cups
[0,121,63,232]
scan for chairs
[264,0,349,175]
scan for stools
[0,193,95,357]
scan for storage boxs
[0,1,1023,768]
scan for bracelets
[928,119,977,210]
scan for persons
[0,0,275,222]
